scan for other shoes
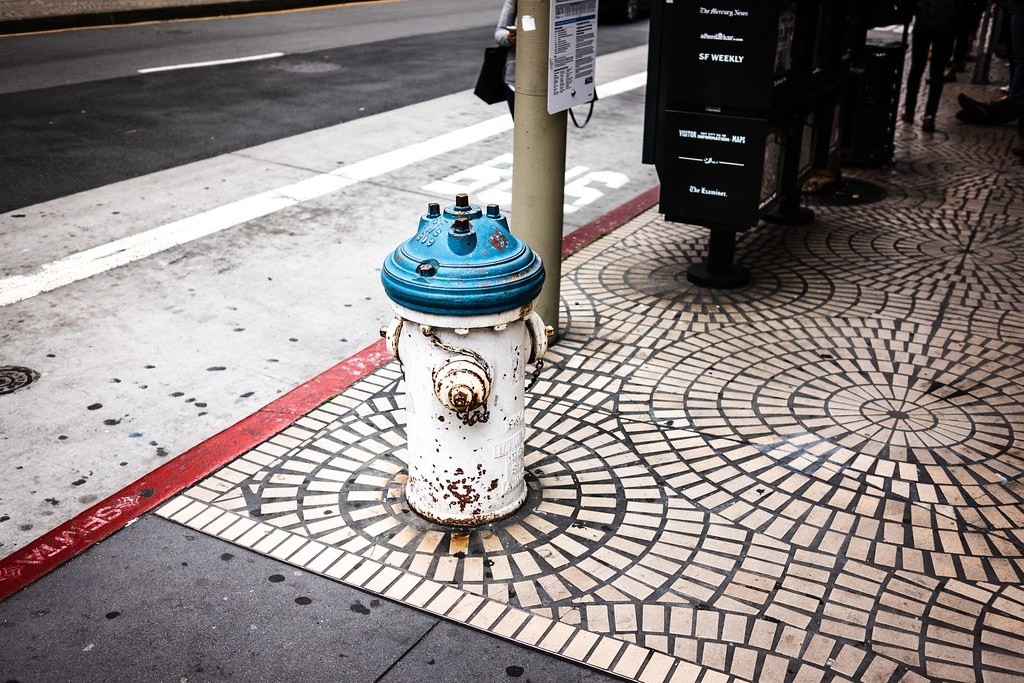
[955,93,994,126]
[925,74,957,84]
[920,119,935,133]
[951,66,965,73]
[901,112,915,124]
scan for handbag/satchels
[473,46,507,104]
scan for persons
[903,0,1024,156]
[495,0,518,121]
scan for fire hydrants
[378,191,556,529]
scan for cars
[597,0,667,25]
